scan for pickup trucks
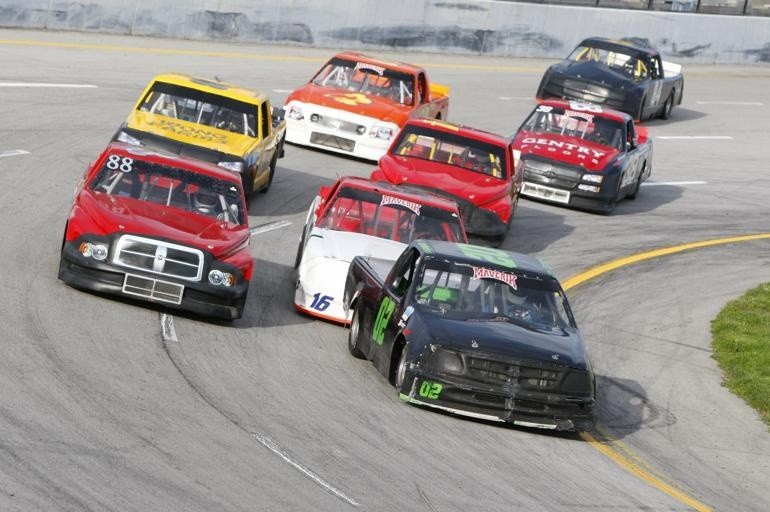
[118,74,286,194]
[291,175,468,328]
[56,142,253,325]
[533,37,684,123]
[342,239,596,435]
[285,54,450,162]
[371,116,524,247]
[510,97,654,212]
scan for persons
[474,150,496,167]
[177,188,224,220]
[485,284,537,323]
[214,111,249,135]
